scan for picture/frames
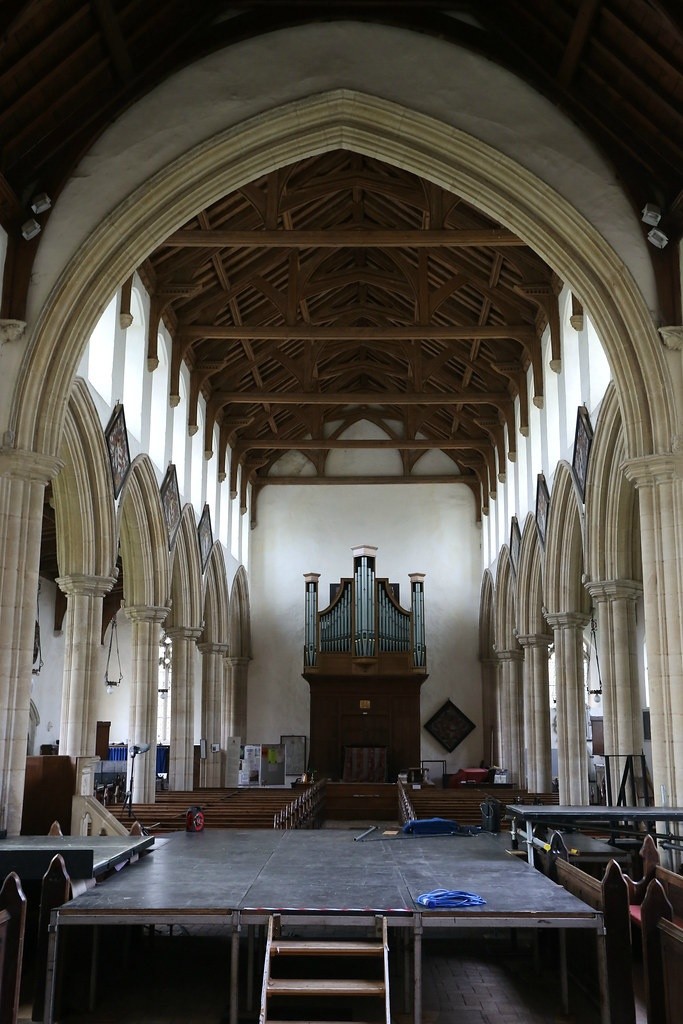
[280,735,306,776]
[423,699,476,753]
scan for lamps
[31,579,45,693]
[585,607,602,702]
[360,698,370,715]
[158,640,172,699]
[104,611,124,694]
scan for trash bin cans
[481,803,500,833]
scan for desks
[0,835,156,883]
[506,804,683,867]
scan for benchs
[396,778,560,831]
[95,786,318,831]
